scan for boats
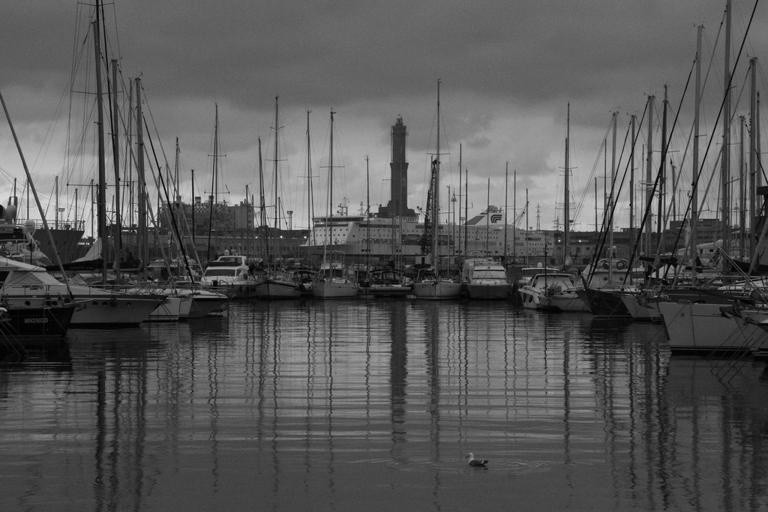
[174,254,268,302]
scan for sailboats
[1,0,231,331]
[410,186,463,297]
[253,94,301,302]
[519,1,767,323]
[358,155,413,297]
[466,159,512,297]
[307,106,363,298]
[639,54,768,353]
[0,99,83,362]
